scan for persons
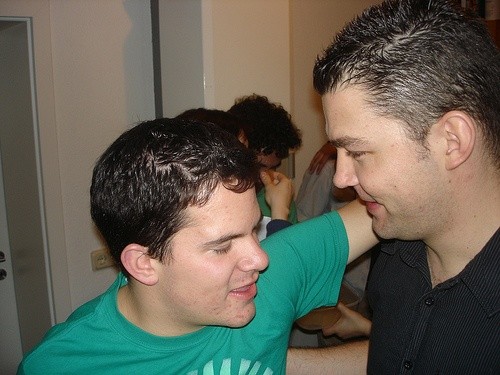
[15,92,381,375]
[286,0,500,375]
